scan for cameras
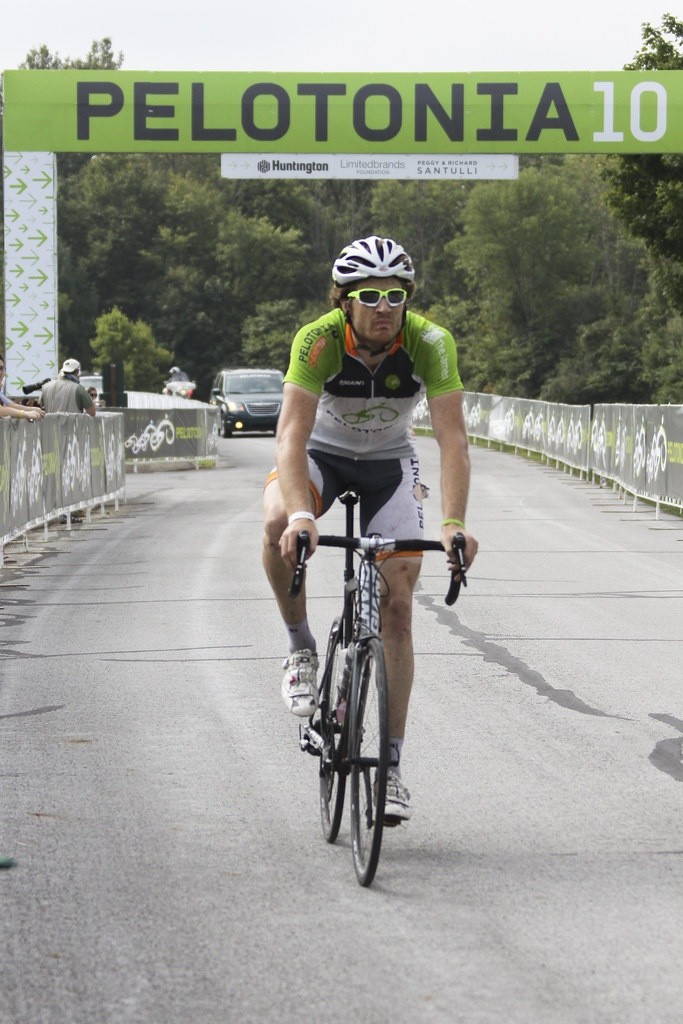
[22,378,51,395]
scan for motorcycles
[164,369,198,399]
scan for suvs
[208,368,287,438]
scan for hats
[62,358,81,373]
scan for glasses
[90,393,97,398]
[347,287,408,307]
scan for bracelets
[441,518,466,529]
[287,510,316,525]
[19,410,24,417]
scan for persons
[83,386,105,412]
[0,354,46,422]
[168,366,190,383]
[39,358,96,416]
[260,234,479,821]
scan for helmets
[332,235,415,289]
[169,366,180,374]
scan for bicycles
[288,494,468,888]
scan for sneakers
[373,769,414,820]
[280,647,320,716]
